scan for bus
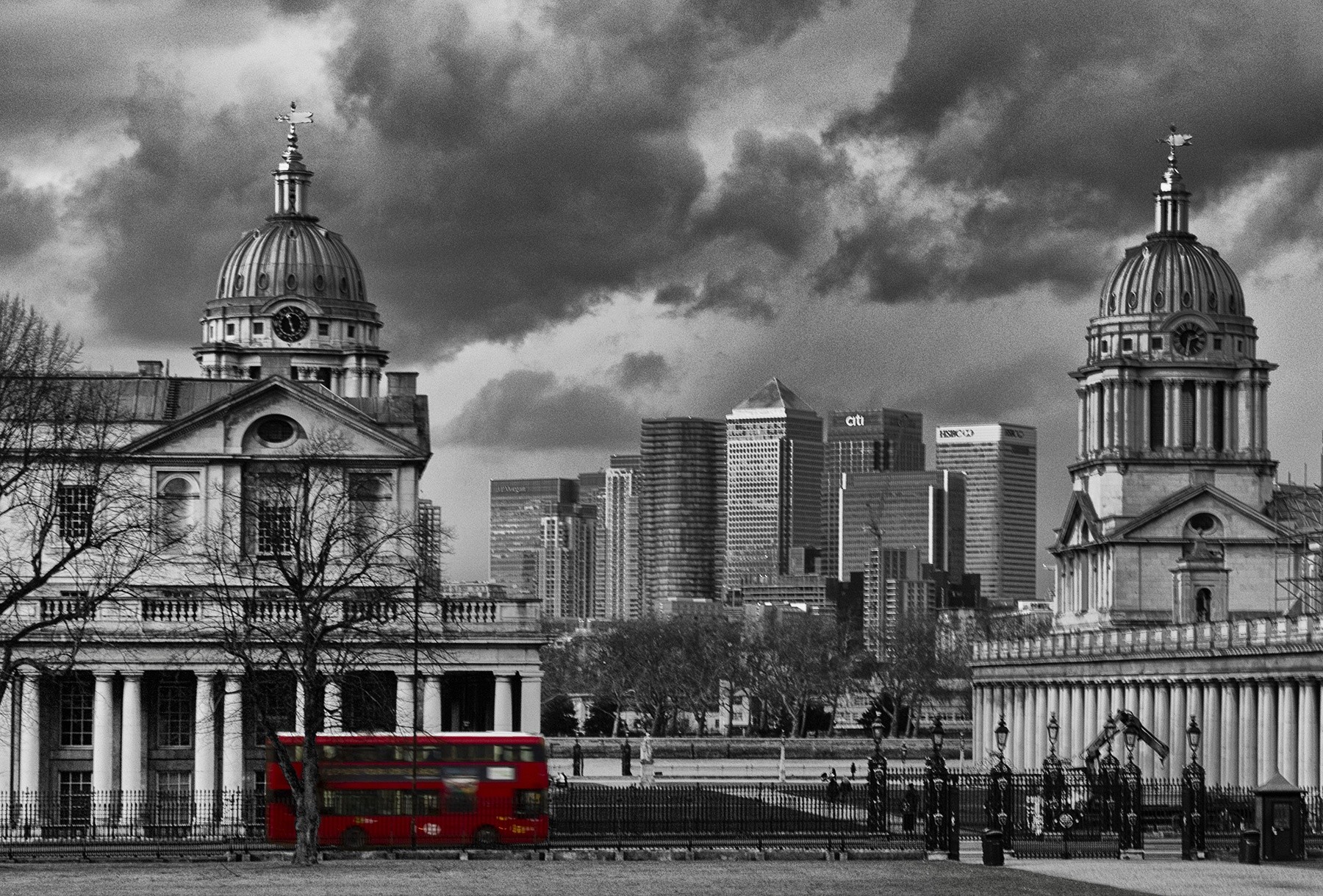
[265,729,555,852]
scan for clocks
[272,305,310,342]
[1173,322,1207,357]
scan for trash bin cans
[1241,828,1260,864]
[980,829,1004,866]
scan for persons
[573,738,584,776]
[621,739,632,776]
[640,736,655,785]
[826,776,921,829]
